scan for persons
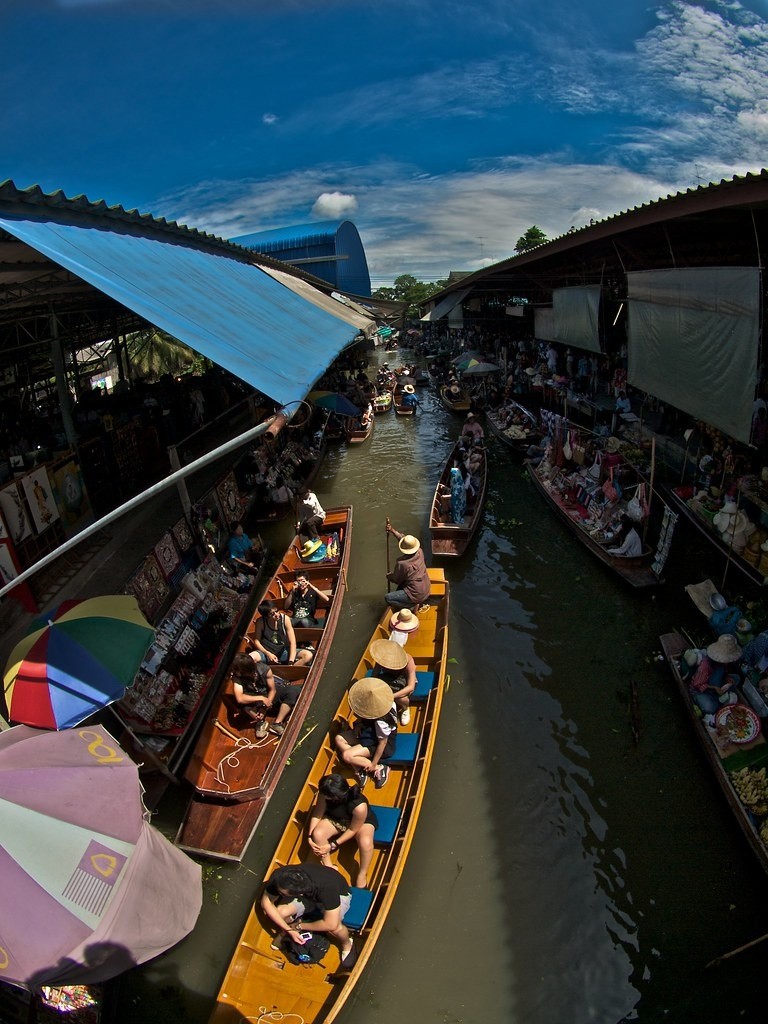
[341,363,460,430]
[231,488,431,969]
[385,328,496,364]
[691,630,768,727]
[495,336,627,398]
[449,389,642,556]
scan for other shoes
[268,720,284,736]
[400,706,410,726]
[341,939,356,967]
[271,935,282,949]
[356,872,366,888]
[254,719,268,738]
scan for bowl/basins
[715,703,761,744]
[709,593,726,610]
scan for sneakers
[353,767,367,788]
[374,764,391,788]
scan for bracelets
[308,835,311,838]
[333,840,339,846]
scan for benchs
[724,744,768,774]
[214,558,434,927]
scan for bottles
[299,954,311,963]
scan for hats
[398,534,420,555]
[707,634,742,664]
[368,638,408,670]
[302,539,322,557]
[464,412,479,422]
[526,367,536,376]
[713,499,756,553]
[448,370,453,375]
[450,385,459,394]
[347,677,394,719]
[404,384,415,394]
[390,607,420,633]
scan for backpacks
[282,923,331,970]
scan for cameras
[297,582,302,586]
[300,933,312,940]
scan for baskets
[744,546,768,573]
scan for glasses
[265,609,279,616]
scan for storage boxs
[691,498,719,524]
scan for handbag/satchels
[563,430,650,523]
[291,593,310,618]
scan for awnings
[0,207,361,594]
[254,263,379,339]
[419,289,471,321]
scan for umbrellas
[453,352,497,377]
[309,391,362,416]
[3,593,157,747]
[0,725,150,984]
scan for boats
[345,343,474,446]
[522,445,685,592]
[659,614,768,876]
[205,561,450,1024]
[429,430,490,561]
[173,503,354,864]
[477,394,548,452]
